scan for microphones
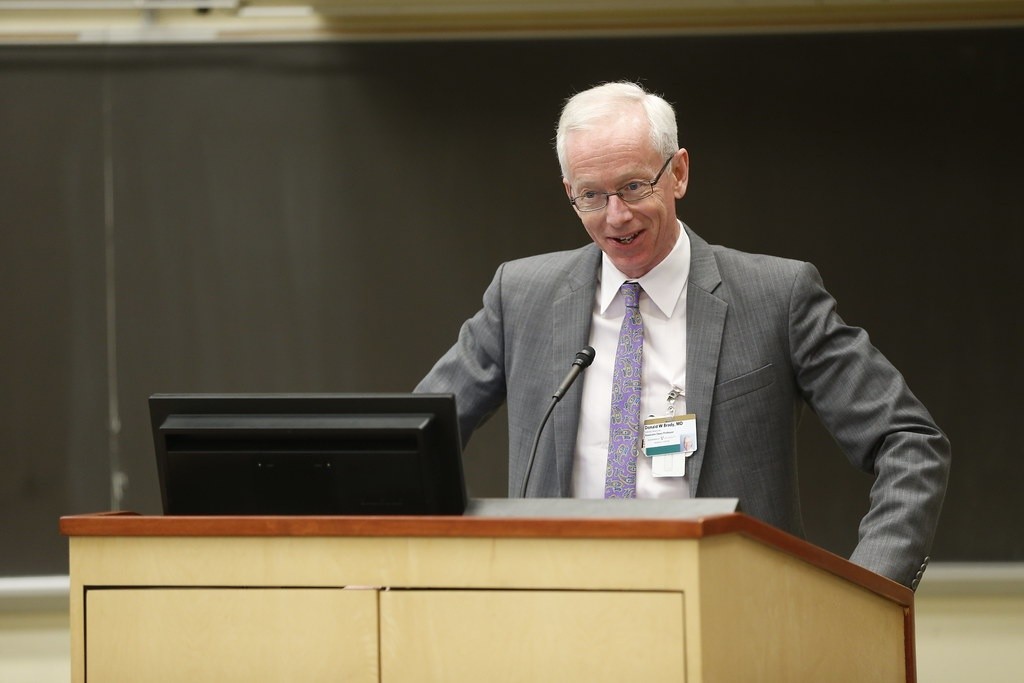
[519,346,596,499]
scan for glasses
[568,152,677,212]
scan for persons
[410,83,952,594]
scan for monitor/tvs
[147,392,469,518]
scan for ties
[605,284,643,499]
